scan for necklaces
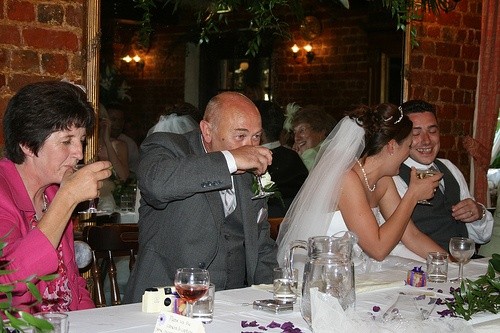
[34,194,47,221]
[358,161,375,192]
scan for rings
[471,212,473,216]
[434,189,436,192]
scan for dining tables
[0,257,500,333]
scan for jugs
[284,230,358,331]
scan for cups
[120,195,134,212]
[272,267,298,303]
[193,283,215,324]
[426,250,449,283]
[35,312,69,333]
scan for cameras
[253,299,294,314]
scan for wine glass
[174,267,210,318]
[72,164,107,214]
[245,168,275,200]
[448,237,475,284]
[414,169,438,205]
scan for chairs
[81,223,140,307]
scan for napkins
[310,287,356,333]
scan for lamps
[304,44,313,62]
[123,55,132,71]
[133,55,144,72]
[291,44,299,58]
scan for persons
[120,91,283,305]
[98,85,201,212]
[0,80,113,320]
[252,100,339,218]
[275,102,452,265]
[392,99,494,263]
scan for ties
[429,165,444,195]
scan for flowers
[283,101,302,132]
[250,172,280,199]
